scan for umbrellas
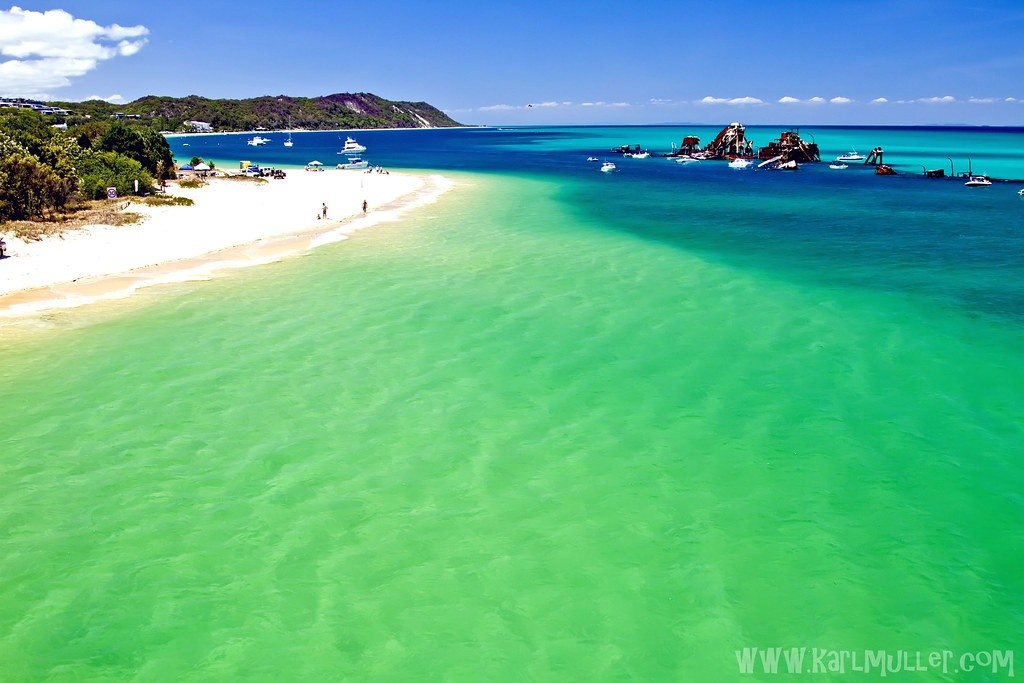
[308,160,324,165]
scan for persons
[363,164,390,175]
[317,214,321,220]
[362,200,368,216]
[305,164,323,172]
[322,203,328,219]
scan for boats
[336,136,367,156]
[247,135,271,147]
[283,138,294,147]
[336,157,368,169]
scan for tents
[178,163,211,180]
